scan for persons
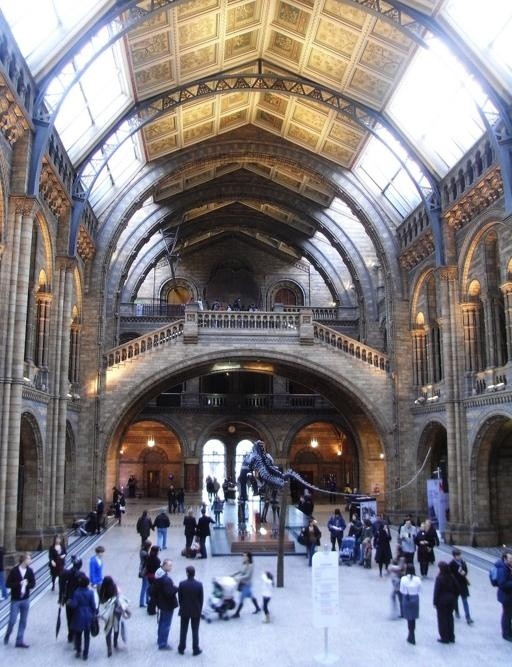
[230,550,274,624]
[433,549,475,644]
[494,547,512,643]
[298,489,440,645]
[73,475,137,536]
[139,540,204,656]
[187,298,261,312]
[3,533,128,661]
[135,476,237,559]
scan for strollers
[199,569,246,624]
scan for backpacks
[489,567,499,588]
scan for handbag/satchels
[90,615,100,637]
[120,620,127,643]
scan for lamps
[311,437,318,448]
[147,435,155,448]
